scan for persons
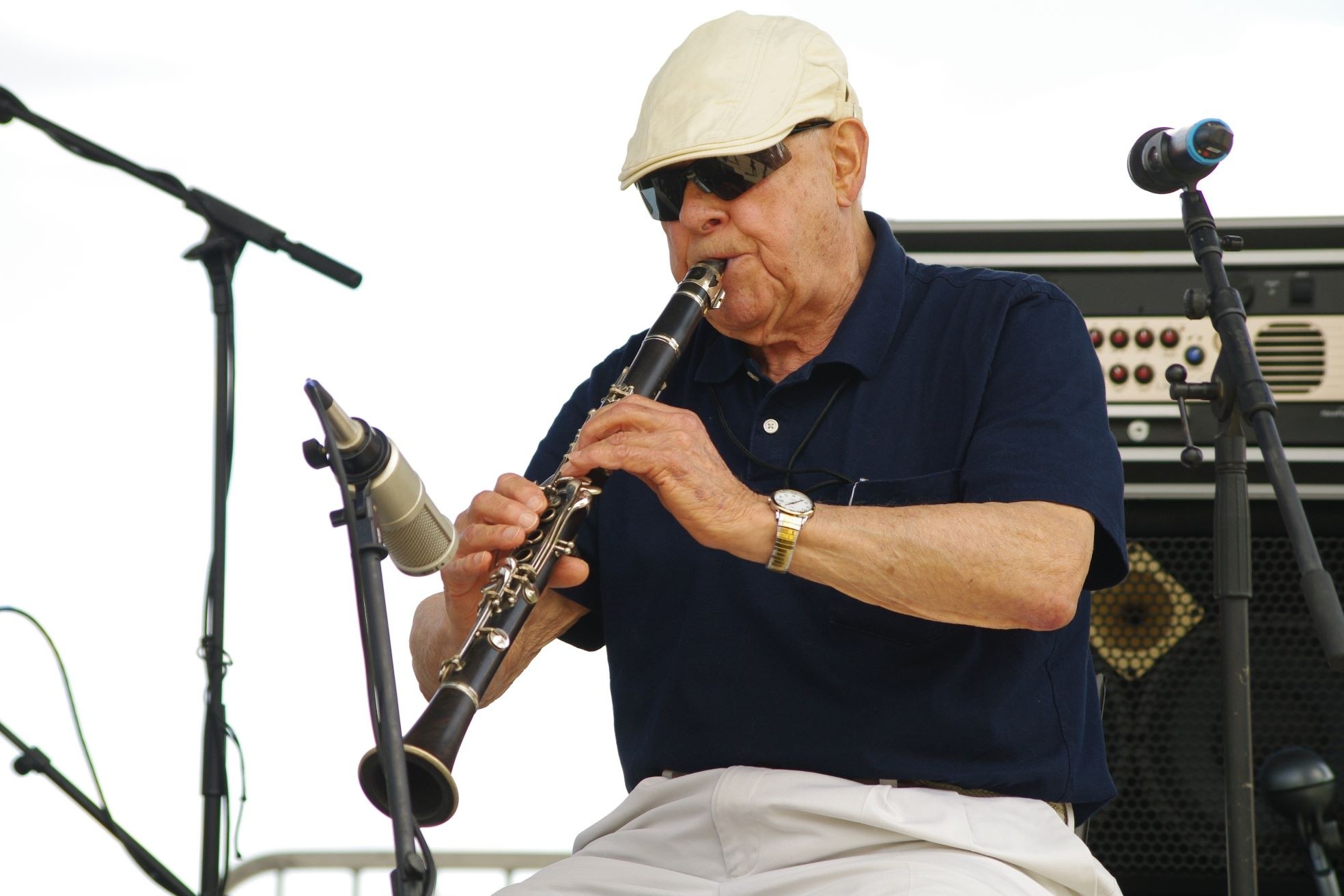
[407,13,1127,896]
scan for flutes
[356,256,731,826]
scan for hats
[619,11,863,192]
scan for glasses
[634,142,793,222]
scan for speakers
[1070,481,1344,896]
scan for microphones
[1127,117,1235,194]
[304,378,458,578]
[1260,747,1336,823]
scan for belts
[647,762,1078,838]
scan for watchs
[766,489,816,573]
